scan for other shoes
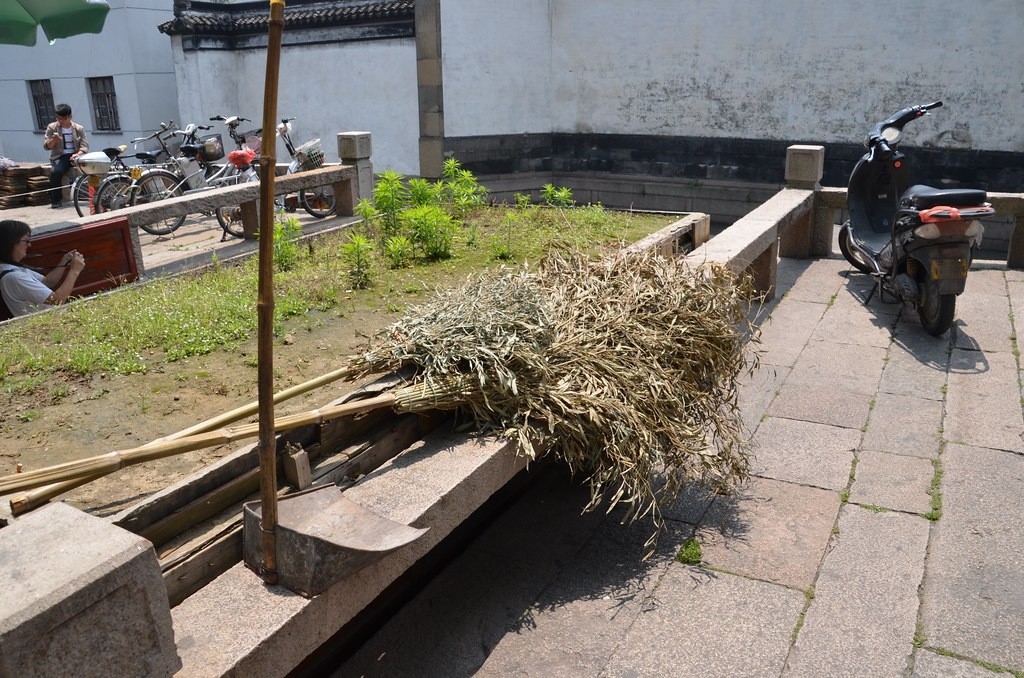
[51,202,62,208]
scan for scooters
[839,99,997,338]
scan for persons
[43,103,89,208]
[0,219,86,317]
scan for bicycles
[69,111,336,240]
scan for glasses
[20,239,30,244]
[56,116,69,121]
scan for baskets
[239,128,262,141]
[196,133,225,161]
[295,138,325,167]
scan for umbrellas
[0,0,114,47]
